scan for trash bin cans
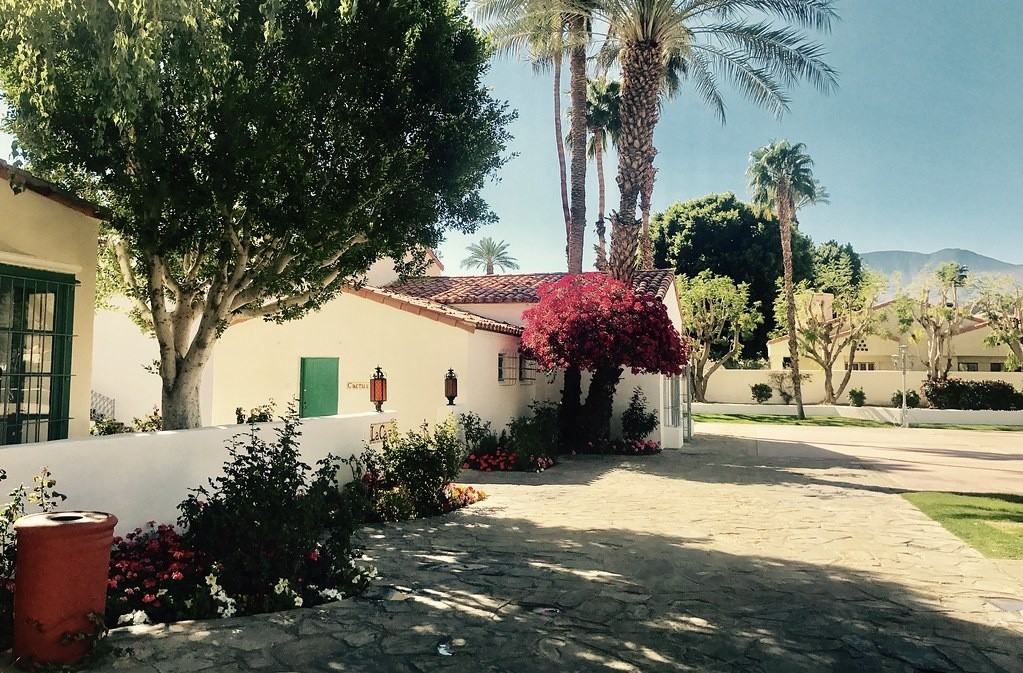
[12,510,120,666]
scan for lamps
[369,364,388,413]
[443,367,458,405]
